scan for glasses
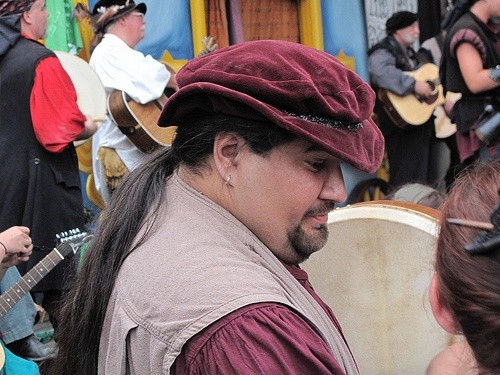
[129,11,144,21]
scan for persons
[0,0,500,375]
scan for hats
[155,38,387,175]
[91,0,147,30]
[386,11,421,32]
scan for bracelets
[0,241,8,255]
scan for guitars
[378,62,448,129]
[1,227,92,339]
[432,104,459,140]
[106,34,220,155]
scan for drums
[297,198,468,375]
